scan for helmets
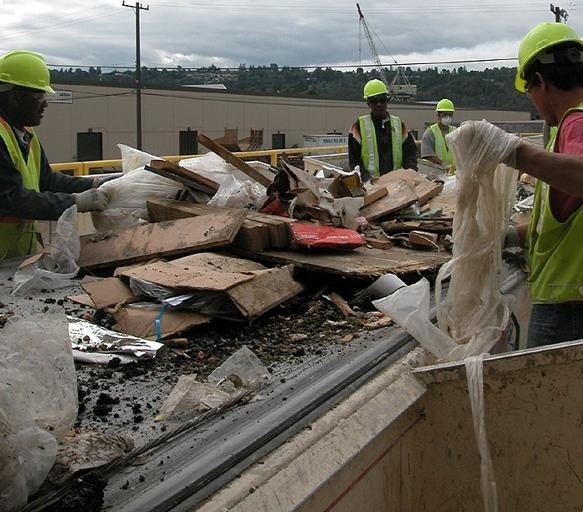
[514,21,583,94]
[0,49,57,95]
[435,99,456,112]
[363,79,390,99]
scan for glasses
[438,111,453,116]
[369,97,389,103]
[10,89,50,104]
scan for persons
[421,99,458,171]
[0,49,110,260]
[348,77,418,183]
[498,22,583,348]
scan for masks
[441,116,454,126]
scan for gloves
[72,189,110,212]
[91,172,124,189]
